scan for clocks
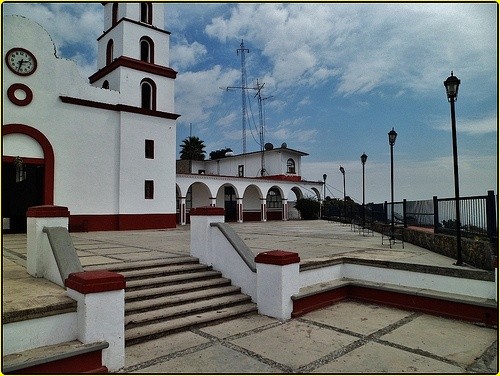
[5,48,37,76]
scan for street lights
[388,127,398,241]
[443,71,464,267]
[360,152,368,229]
[339,166,349,223]
[323,174,327,220]
